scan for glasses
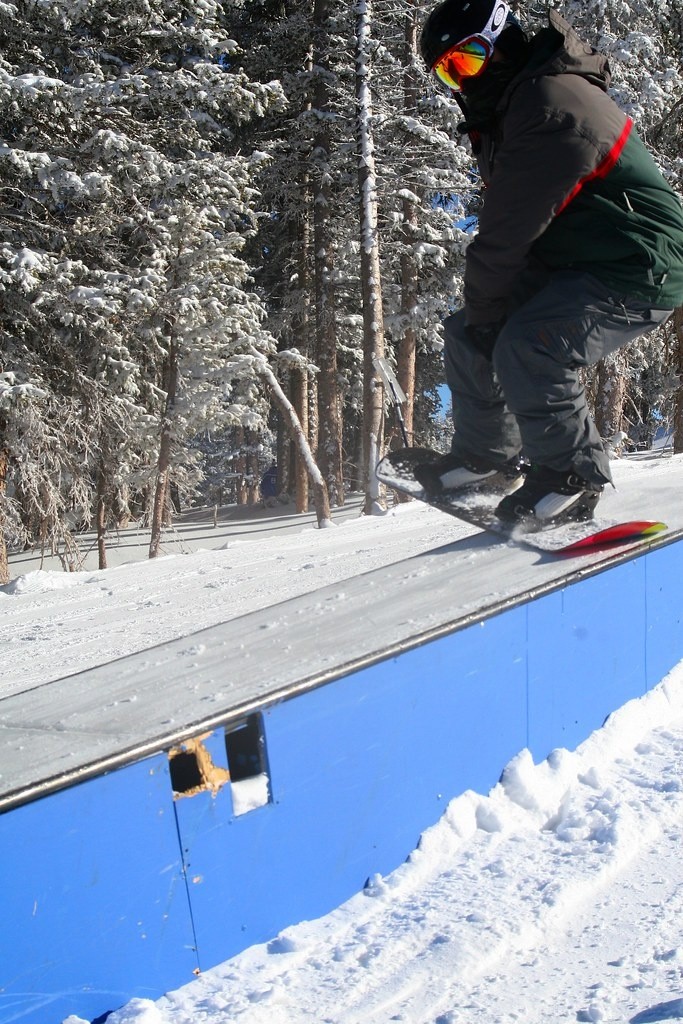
[431,34,496,92]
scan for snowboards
[375,447,670,553]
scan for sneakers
[414,447,517,498]
[494,470,603,532]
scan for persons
[412,0,682,525]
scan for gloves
[462,294,508,364]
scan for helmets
[420,0,527,97]
